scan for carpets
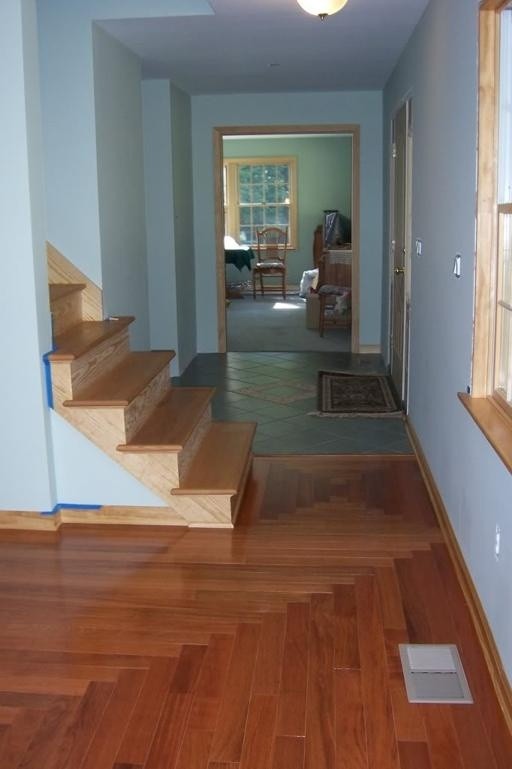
[316,368,402,419]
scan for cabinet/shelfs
[306,225,351,329]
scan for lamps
[296,0,347,22]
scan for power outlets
[492,525,503,563]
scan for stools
[319,285,351,337]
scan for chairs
[251,226,288,300]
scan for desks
[224,247,253,271]
